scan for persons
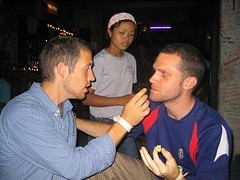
[81,12,141,160]
[0,35,151,180]
[0,76,11,111]
[135,39,181,85]
[76,42,233,180]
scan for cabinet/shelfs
[17,10,79,72]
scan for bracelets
[115,115,133,133]
[176,166,189,180]
